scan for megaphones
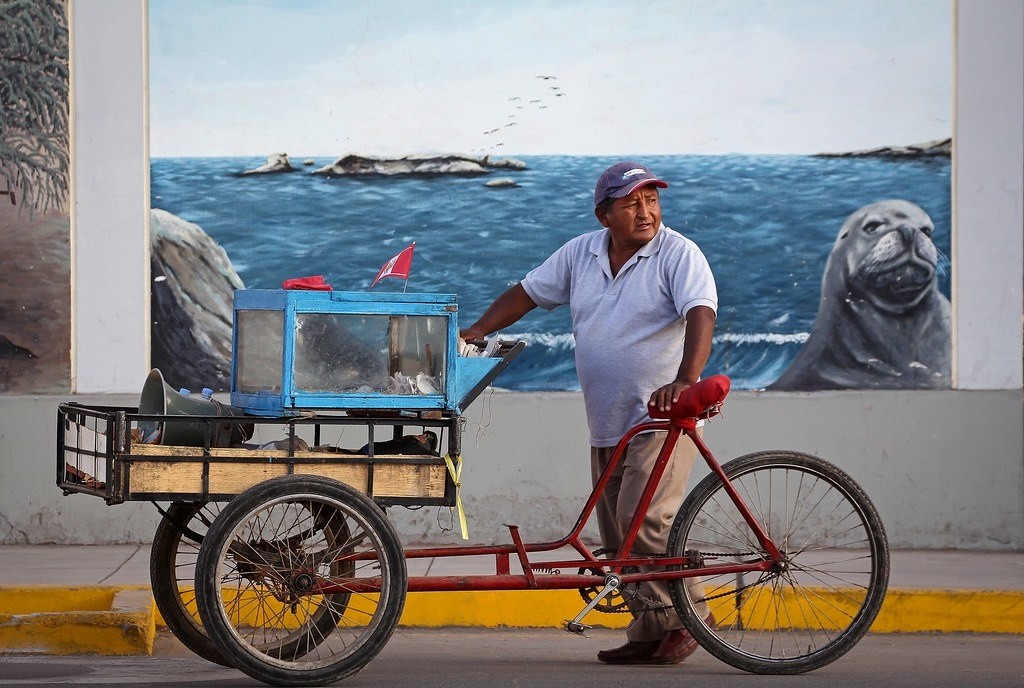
[137,367,255,448]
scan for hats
[594,160,669,208]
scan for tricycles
[57,338,890,688]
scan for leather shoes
[652,611,714,664]
[597,638,661,665]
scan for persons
[459,163,718,664]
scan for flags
[371,244,414,287]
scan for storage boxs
[229,290,459,414]
